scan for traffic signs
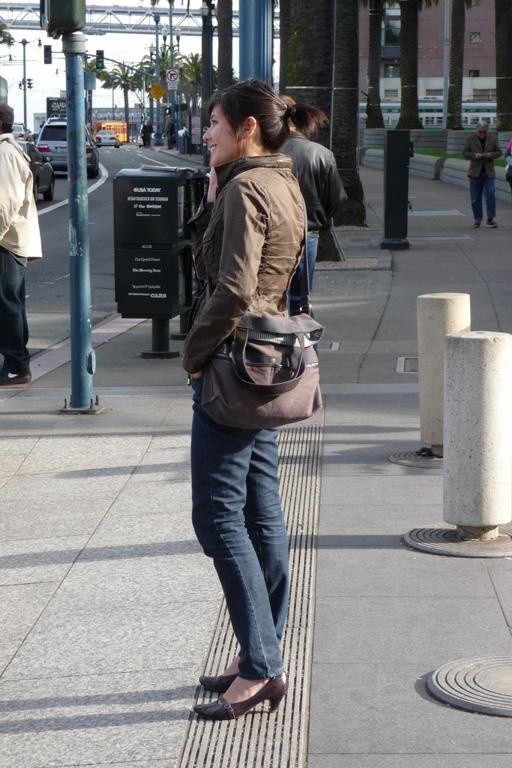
[167,82,178,91]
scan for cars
[11,124,30,142]
[14,140,56,205]
[95,130,121,149]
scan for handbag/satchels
[195,284,326,430]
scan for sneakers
[485,221,498,228]
[0,368,32,385]
[472,222,480,228]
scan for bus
[374,96,497,130]
[90,118,129,146]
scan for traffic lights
[38,0,87,35]
[96,48,104,69]
[43,44,53,64]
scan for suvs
[33,117,99,178]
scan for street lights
[17,38,33,132]
[152,13,165,144]
[163,2,182,148]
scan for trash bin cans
[112,164,210,319]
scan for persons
[503,135,512,194]
[180,75,312,721]
[462,119,503,229]
[0,101,44,389]
[163,117,177,151]
[273,94,349,361]
[140,120,153,146]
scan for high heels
[198,669,239,691]
[194,673,288,719]
